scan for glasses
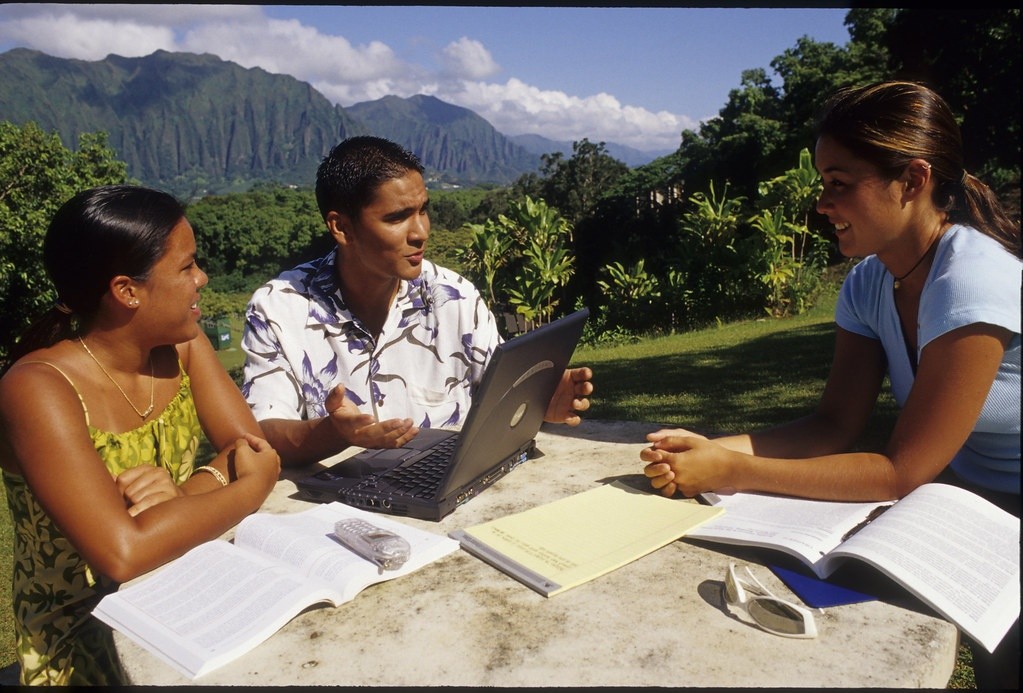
[722,563,817,639]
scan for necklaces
[892,239,939,289]
[76,333,155,418]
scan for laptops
[298,306,591,523]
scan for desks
[104,417,961,691]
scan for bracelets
[189,465,227,487]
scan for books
[446,479,724,599]
[91,502,461,677]
[682,482,1023,655]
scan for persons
[641,83,1023,693]
[0,185,282,686]
[238,136,593,470]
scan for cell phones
[335,519,411,570]
